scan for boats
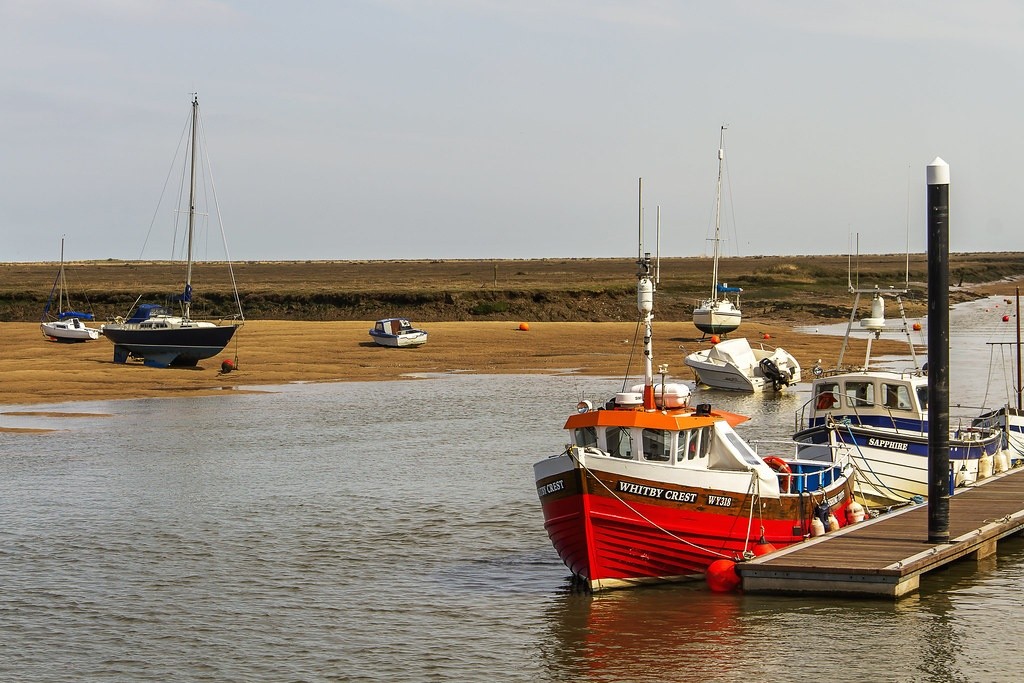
[368,317,429,348]
[683,332,802,395]
[968,283,1024,470]
[787,222,1004,516]
[529,178,864,597]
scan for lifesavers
[763,456,793,493]
[116,316,124,325]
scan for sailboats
[691,123,745,339]
[103,89,247,369]
[39,236,104,344]
